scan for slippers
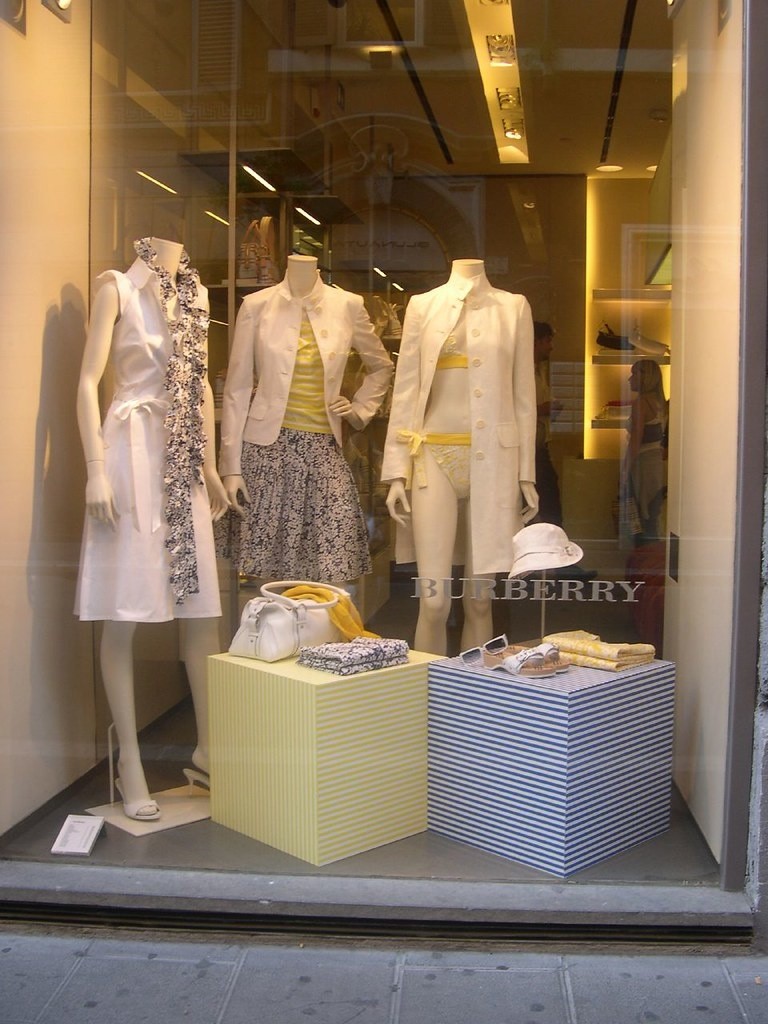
[505,642,569,673]
[483,646,556,678]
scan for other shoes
[556,564,597,581]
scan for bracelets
[621,471,628,474]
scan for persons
[72,236,232,820]
[212,254,394,597]
[619,360,669,548]
[534,321,598,583]
[380,259,540,656]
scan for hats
[508,523,584,582]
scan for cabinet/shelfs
[179,0,392,626]
[591,288,672,429]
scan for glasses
[459,634,509,666]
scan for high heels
[113,778,161,821]
[182,768,212,797]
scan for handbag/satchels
[227,581,350,664]
[637,397,663,444]
[612,491,642,535]
